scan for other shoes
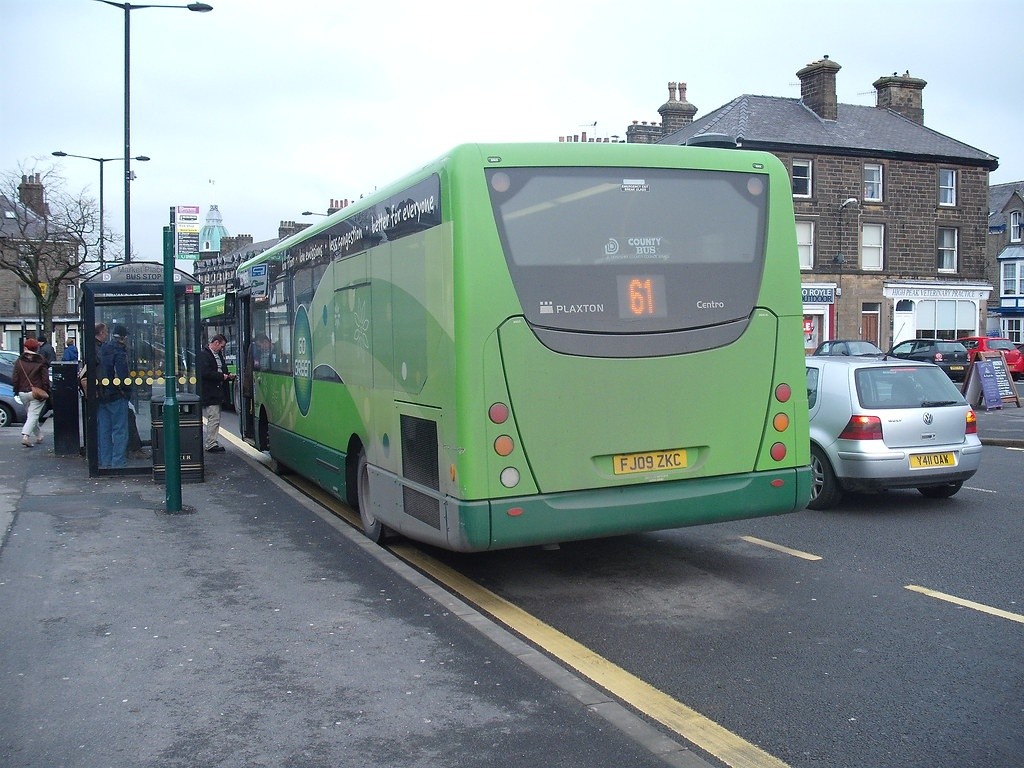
[22,438,34,447]
[205,445,225,453]
[36,436,44,444]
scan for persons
[61,338,78,361]
[37,337,56,364]
[95,323,151,468]
[11,339,50,447]
[195,334,237,452]
[253,333,283,369]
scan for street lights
[53,151,151,325]
[96,0,214,373]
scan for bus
[199,293,237,410]
[223,140,813,555]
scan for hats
[113,326,130,337]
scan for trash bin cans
[150,392,204,484]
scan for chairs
[947,344,954,351]
[890,377,919,403]
[917,344,925,352]
[938,343,946,351]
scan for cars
[956,336,1024,382]
[0,350,54,428]
[805,356,983,512]
[886,338,971,382]
[813,339,885,357]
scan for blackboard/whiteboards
[960,351,1020,402]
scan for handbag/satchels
[31,386,49,402]
